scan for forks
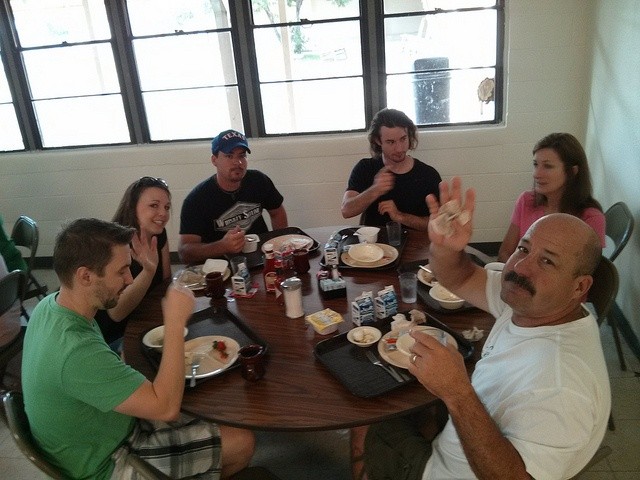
[364,349,403,383]
[190,354,201,387]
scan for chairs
[1,268,29,391]
[1,387,70,479]
[564,257,620,429]
[10,214,48,323]
[603,201,634,372]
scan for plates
[178,334,242,379]
[174,262,230,290]
[378,327,457,370]
[261,234,313,255]
[341,244,399,268]
[417,263,440,288]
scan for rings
[410,354,418,362]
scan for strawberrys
[212,341,226,352]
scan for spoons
[236,229,254,241]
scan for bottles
[280,277,305,319]
[275,268,287,306]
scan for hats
[211,130,251,154]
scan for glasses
[132,177,168,193]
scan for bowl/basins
[483,262,505,275]
[353,225,380,241]
[349,243,384,265]
[241,235,259,253]
[142,324,189,349]
[396,334,424,359]
[283,238,311,250]
[202,257,227,277]
[428,285,466,310]
[347,325,380,348]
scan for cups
[238,343,267,381]
[386,221,401,245]
[230,256,246,275]
[398,272,417,303]
[204,273,225,298]
[422,328,447,349]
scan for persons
[407,175,613,480]
[19,218,257,480]
[177,129,289,266]
[95,176,172,356]
[497,132,606,263]
[341,108,442,231]
